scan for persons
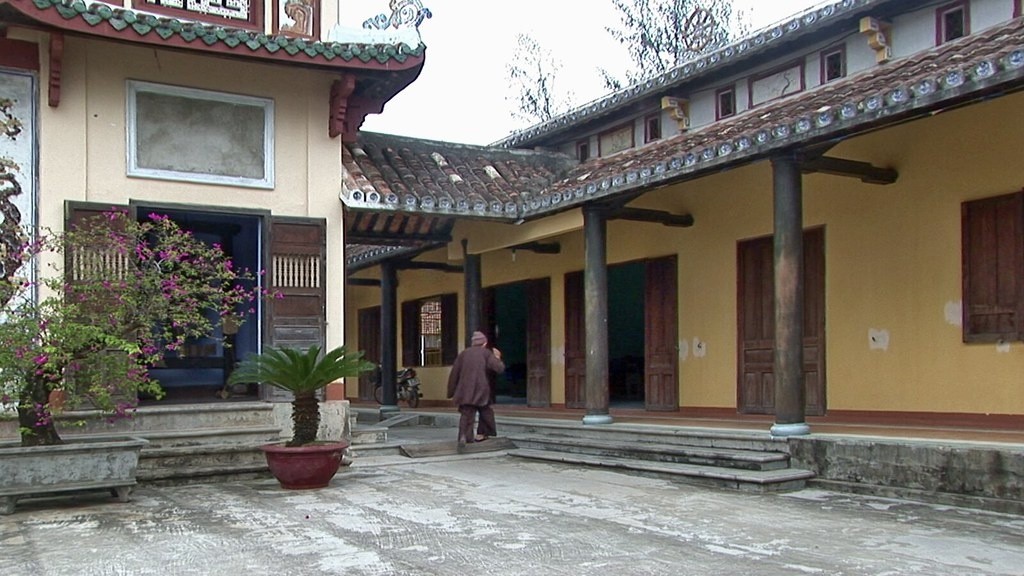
[446,331,505,443]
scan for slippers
[476,434,489,441]
[456,438,466,454]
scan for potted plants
[226,346,377,490]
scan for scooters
[374,356,424,409]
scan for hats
[471,331,487,345]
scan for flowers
[0,207,284,435]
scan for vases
[0,434,154,513]
